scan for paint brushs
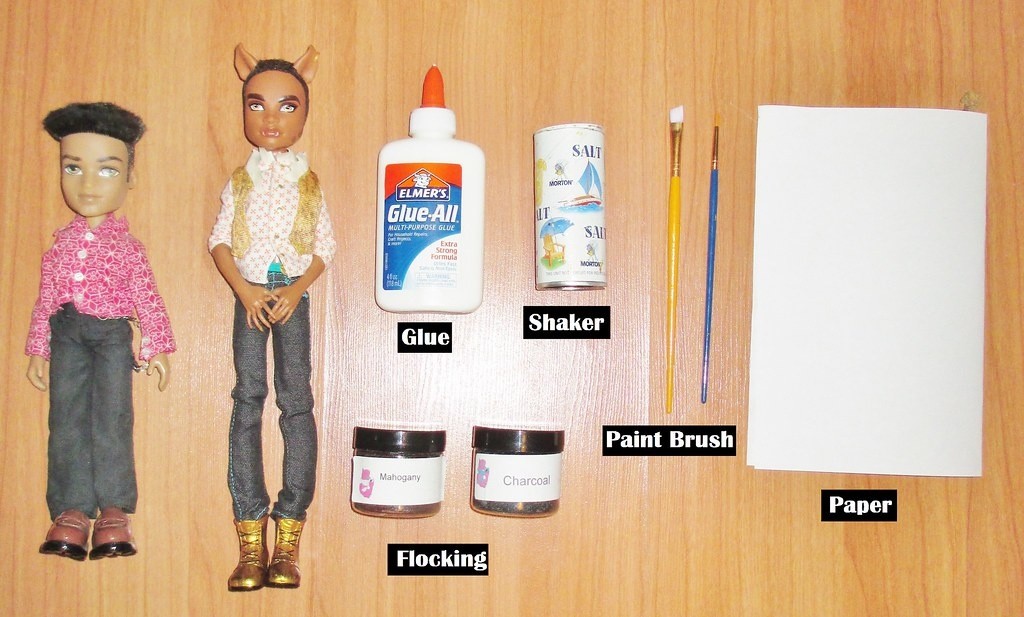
[700,110,718,403]
[664,104,685,415]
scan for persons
[207,43,336,592]
[23,101,178,563]
